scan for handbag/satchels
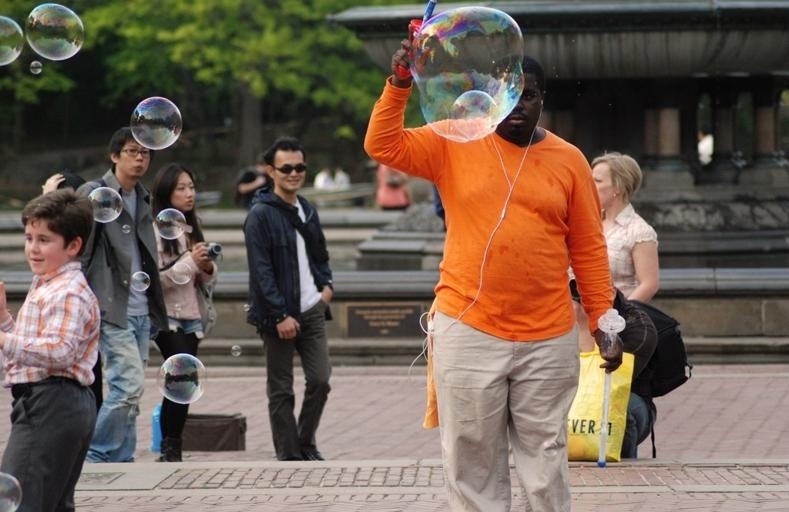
[559,351,635,463]
[195,278,217,337]
[324,305,332,320]
[91,350,103,414]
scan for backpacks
[621,392,657,458]
[569,279,692,398]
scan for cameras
[205,240,223,261]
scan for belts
[25,376,66,386]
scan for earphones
[541,99,544,106]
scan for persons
[235,152,273,208]
[0,189,102,512]
[243,136,332,461]
[313,156,350,190]
[697,124,715,171]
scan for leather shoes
[302,447,325,461]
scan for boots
[155,436,183,462]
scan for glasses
[120,148,149,158]
[273,163,306,173]
